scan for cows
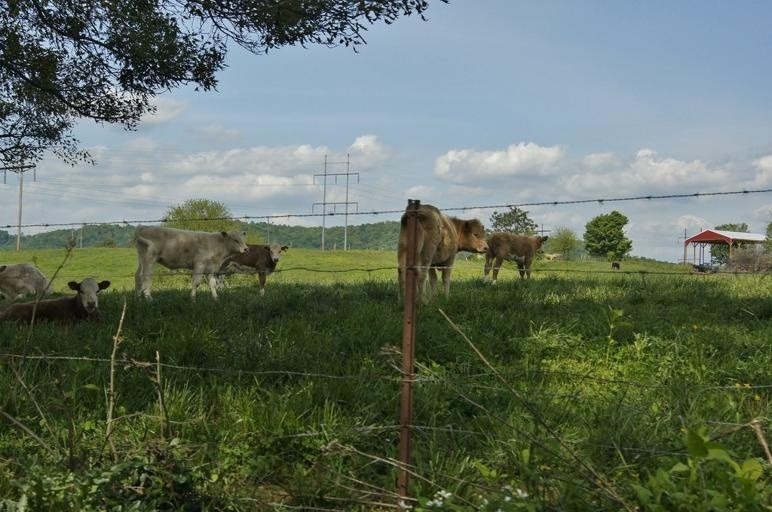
[0,278,111,331]
[482,232,549,286]
[398,203,490,310]
[0,263,51,303]
[134,224,250,304]
[216,243,289,295]
